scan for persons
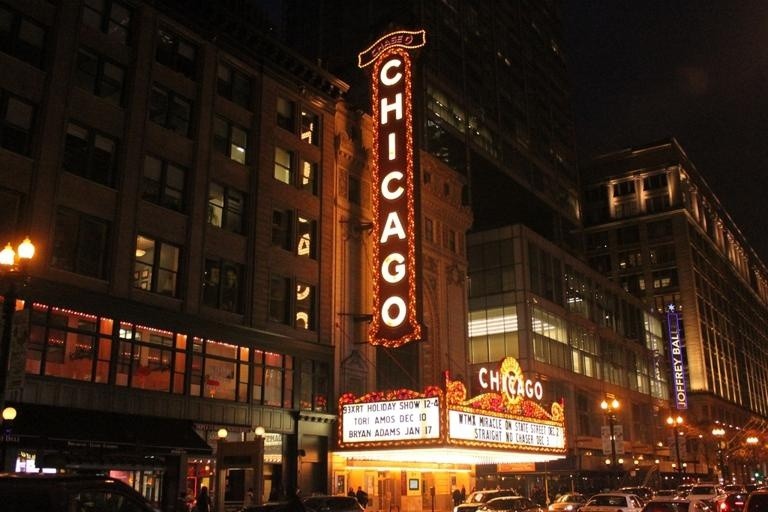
[348,485,366,508]
[454,485,466,507]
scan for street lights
[599,397,621,477]
[0,231,38,442]
[711,428,729,484]
[746,436,761,479]
[0,402,19,473]
[665,415,685,485]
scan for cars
[243,493,367,511]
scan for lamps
[135,249,146,256]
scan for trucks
[0,469,166,512]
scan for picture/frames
[133,269,149,290]
